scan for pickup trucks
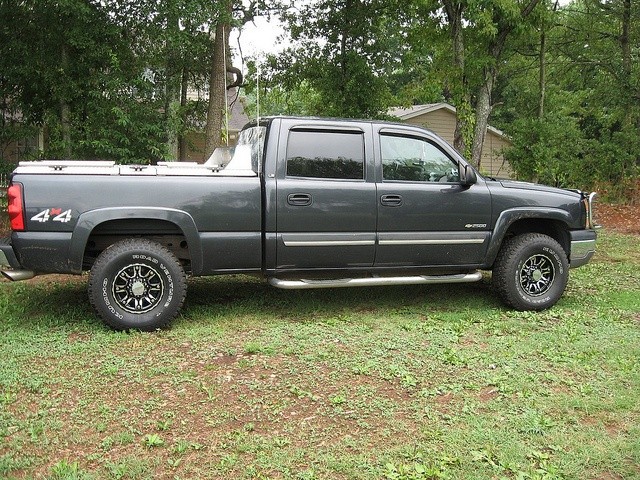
[1,112,598,333]
